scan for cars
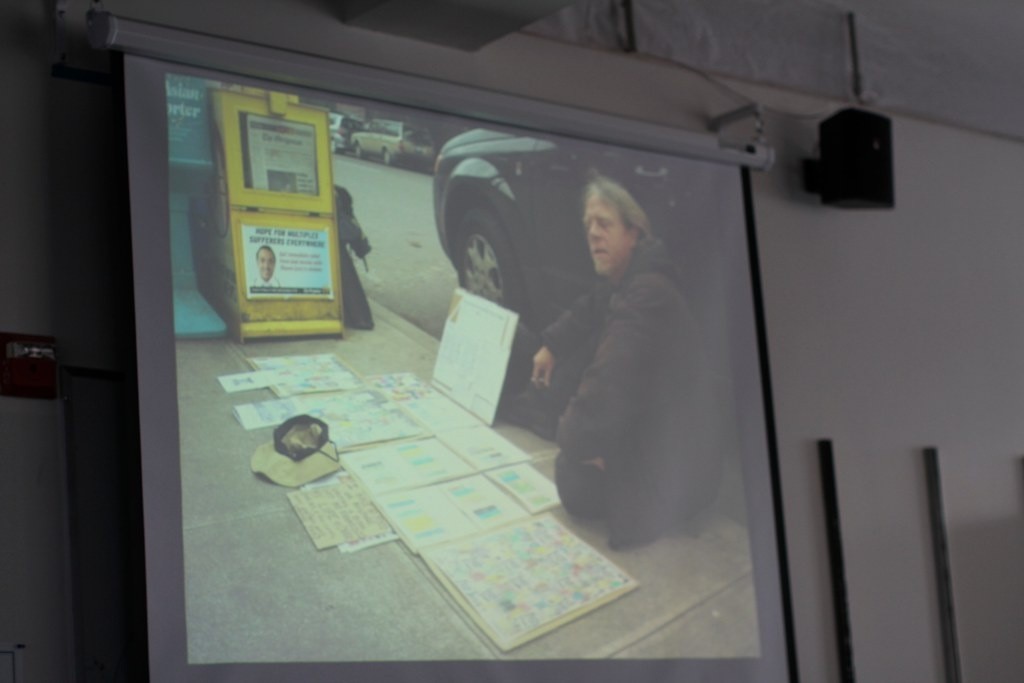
[325,110,684,321]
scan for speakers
[817,107,893,208]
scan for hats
[251,414,341,488]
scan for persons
[531,175,737,546]
[251,247,279,288]
